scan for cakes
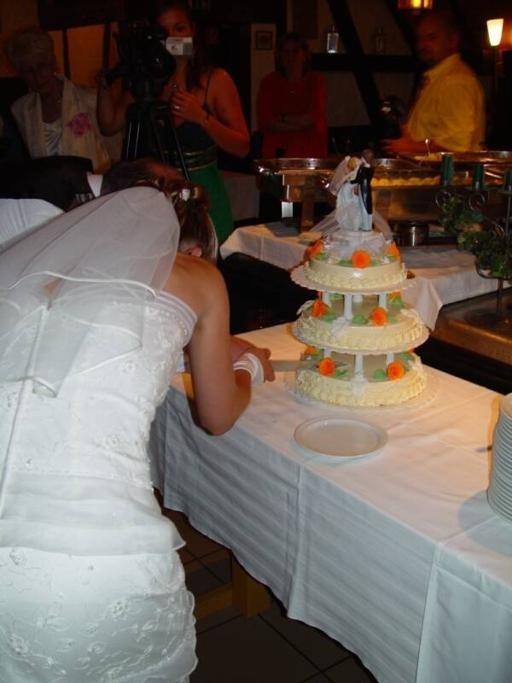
[289,153,431,407]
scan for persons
[0,2,487,244]
[0,152,274,683]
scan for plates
[293,413,389,463]
[487,394,512,521]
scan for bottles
[434,152,455,193]
[326,25,341,54]
[465,161,489,204]
[398,220,430,248]
[374,26,386,56]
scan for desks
[229,221,512,308]
[163,316,512,683]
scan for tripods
[124,98,191,182]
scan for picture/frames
[256,30,272,50]
